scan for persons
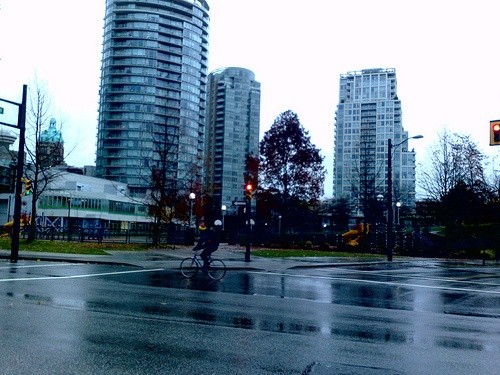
[192,223,218,273]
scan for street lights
[222,204,226,231]
[189,193,196,227]
[396,202,401,225]
[387,135,424,261]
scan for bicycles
[180,248,227,281]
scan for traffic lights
[25,178,32,196]
[245,157,258,172]
[489,120,500,146]
[244,172,258,194]
